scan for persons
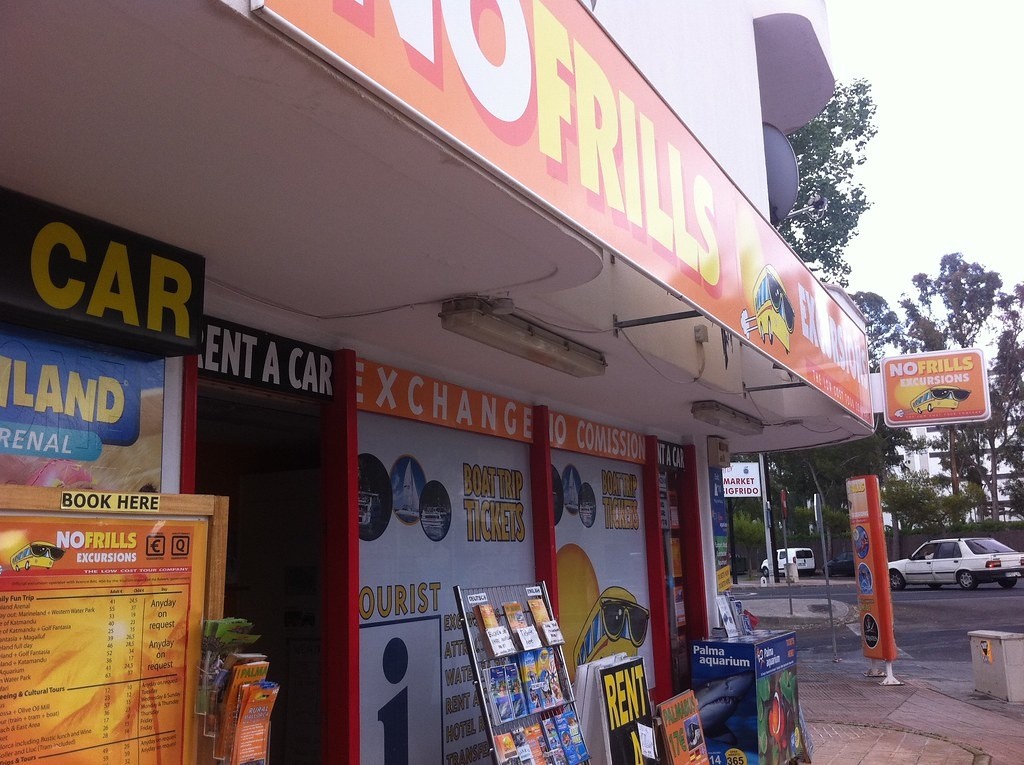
[924,543,938,560]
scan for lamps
[690,400,764,437]
[437,296,607,380]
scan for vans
[760,548,816,578]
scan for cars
[887,536,1024,591]
[822,551,855,577]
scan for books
[727,595,752,639]
[482,648,563,724]
[472,599,550,659]
[494,711,590,765]
[196,617,279,765]
